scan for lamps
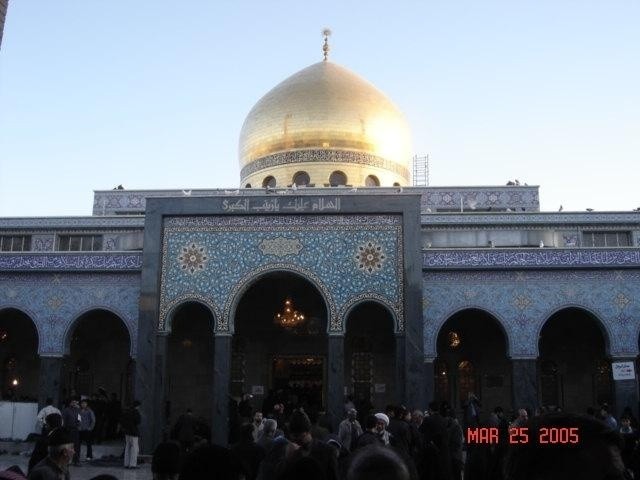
[272,296,306,331]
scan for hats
[374,413,390,427]
[47,428,75,446]
